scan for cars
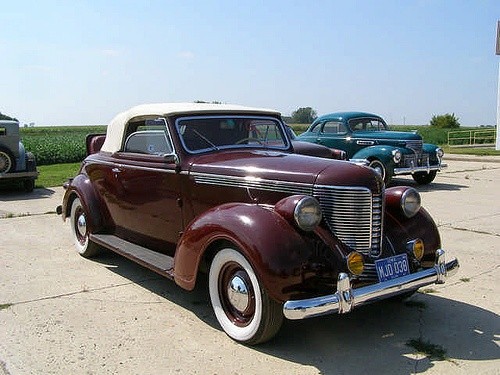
[55,101,461,347]
[1,117,38,193]
[293,108,444,187]
